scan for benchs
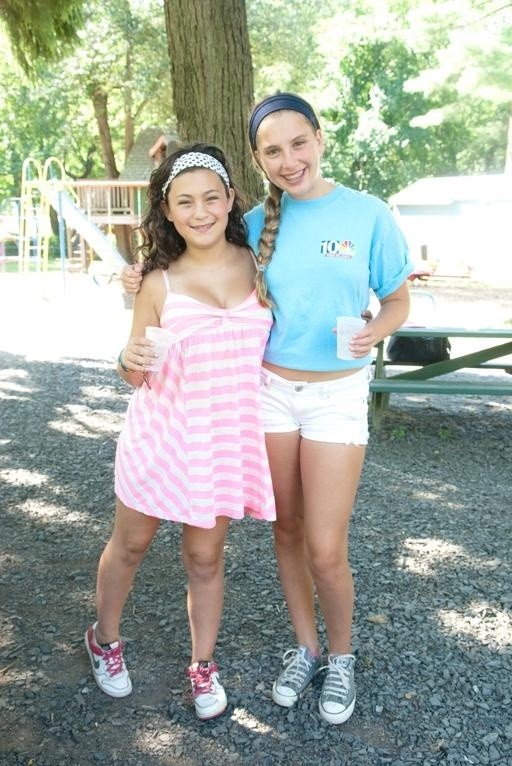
[371,360,512,369]
[369,379,512,394]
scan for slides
[35,182,129,276]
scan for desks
[372,327,512,432]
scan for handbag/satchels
[385,328,451,364]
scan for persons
[118,92,419,727]
[81,144,283,722]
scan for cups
[145,326,174,373]
[336,316,366,360]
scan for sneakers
[271,646,322,707]
[318,653,356,726]
[85,621,133,699]
[184,659,228,720]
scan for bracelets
[118,348,136,375]
